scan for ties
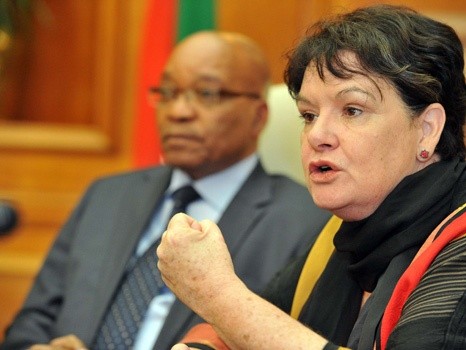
[88,184,203,350]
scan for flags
[131,1,219,171]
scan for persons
[1,27,332,350]
[156,5,465,349]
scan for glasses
[147,83,261,111]
